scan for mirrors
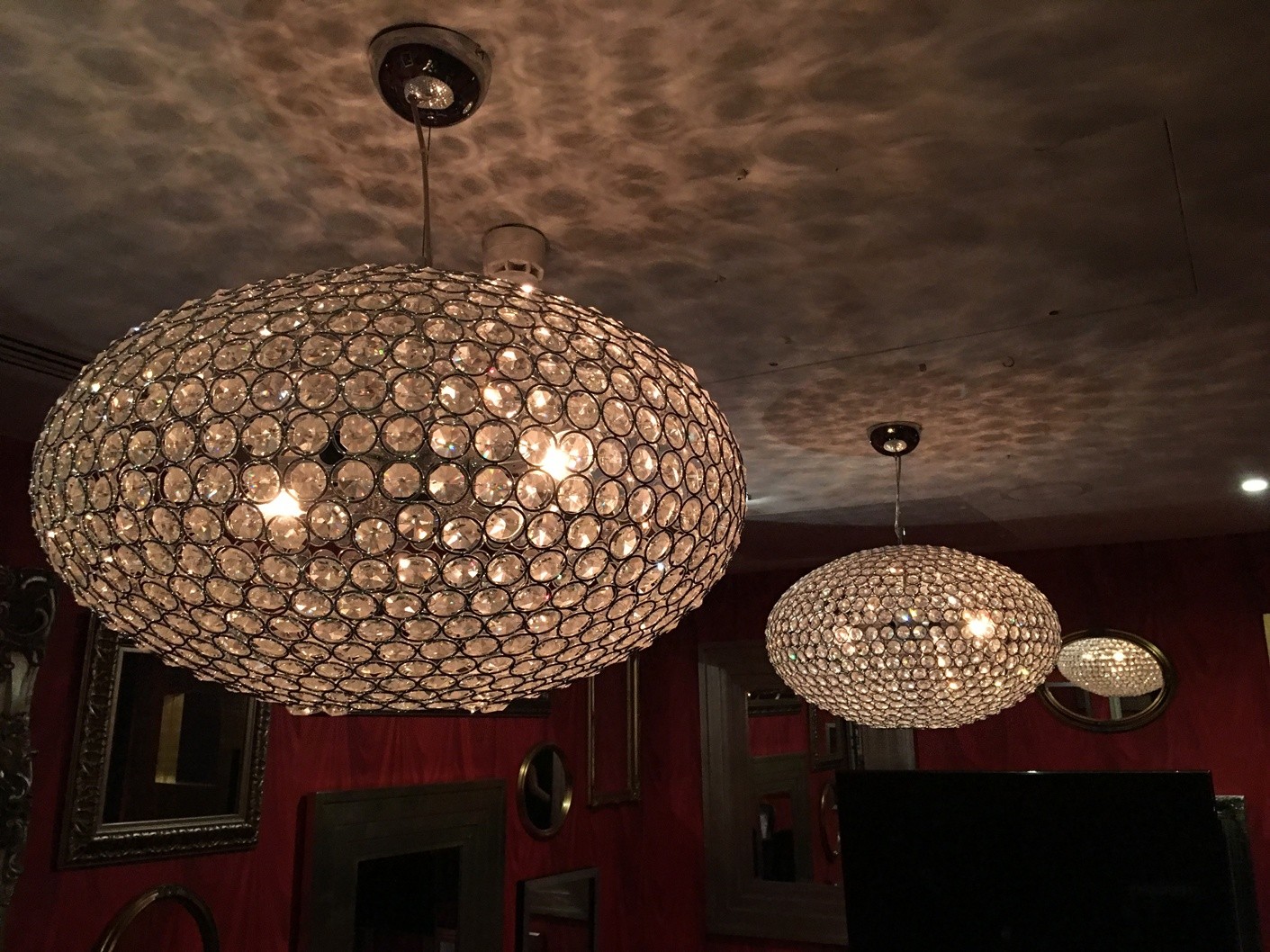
[518,742,575,841]
[809,703,848,770]
[747,675,801,718]
[55,604,270,868]
[696,635,920,945]
[1038,630,1178,732]
[587,656,641,814]
[300,777,506,952]
[753,754,814,882]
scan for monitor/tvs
[835,769,1229,952]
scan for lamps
[1058,638,1164,697]
[46,23,748,720]
[763,421,1064,733]
